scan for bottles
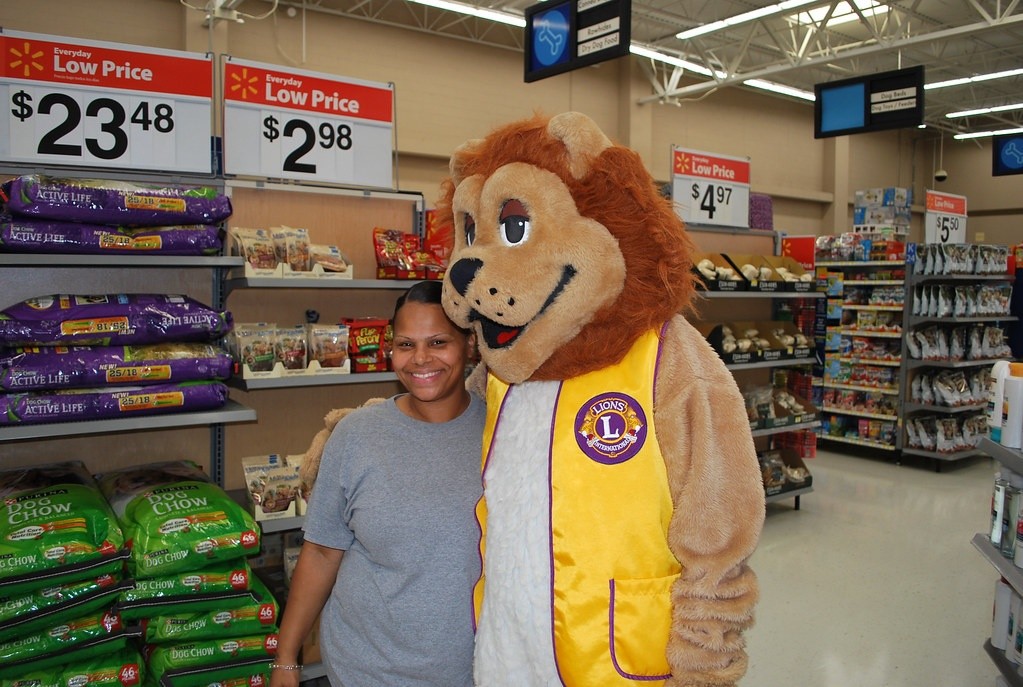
[988,460,1023,572]
[1000,362,1023,449]
[990,574,1023,674]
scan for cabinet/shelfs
[968,436,1023,687]
[690,291,827,510]
[0,251,259,445]
[812,240,908,466]
[895,262,1018,474]
[225,276,446,683]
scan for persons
[268,281,487,687]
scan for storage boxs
[854,187,914,225]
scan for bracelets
[269,663,303,670]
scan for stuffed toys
[299,110,768,687]
